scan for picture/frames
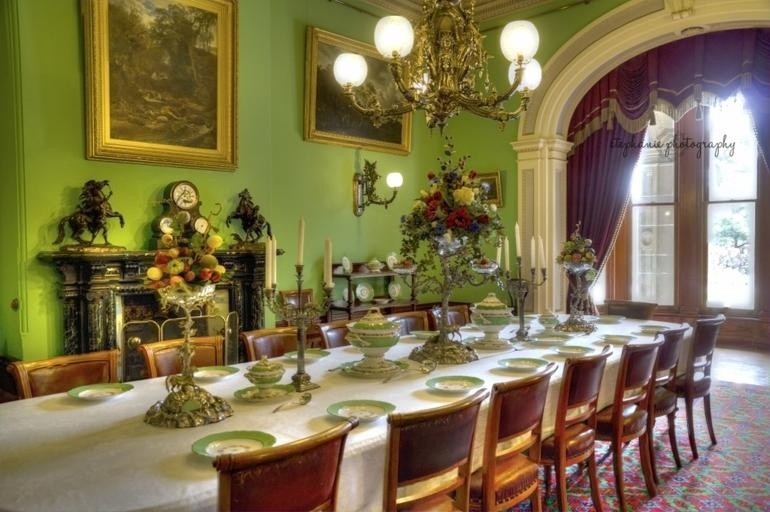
[477,172,504,210]
[81,1,239,170]
[280,289,314,329]
[305,26,412,156]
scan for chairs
[530,344,613,512]
[666,313,726,460]
[450,362,557,512]
[239,325,309,360]
[315,319,359,349]
[7,351,119,398]
[214,416,361,511]
[430,305,469,330]
[138,334,226,379]
[384,310,428,335]
[578,334,664,512]
[648,323,690,485]
[382,388,489,511]
[604,300,657,320]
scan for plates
[284,350,331,363]
[192,365,240,382]
[192,430,277,460]
[426,376,484,394]
[234,384,296,402]
[327,400,396,423]
[555,345,594,356]
[498,357,548,371]
[68,383,134,401]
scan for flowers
[398,135,505,364]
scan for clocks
[152,180,219,251]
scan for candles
[296,216,304,265]
[323,236,332,288]
[265,236,271,289]
[504,236,509,272]
[537,234,546,269]
[514,221,521,257]
[531,235,535,270]
[495,235,502,268]
[271,233,277,288]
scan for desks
[36,245,269,384]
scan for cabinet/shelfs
[323,260,417,323]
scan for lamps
[333,1,542,137]
[352,157,403,219]
